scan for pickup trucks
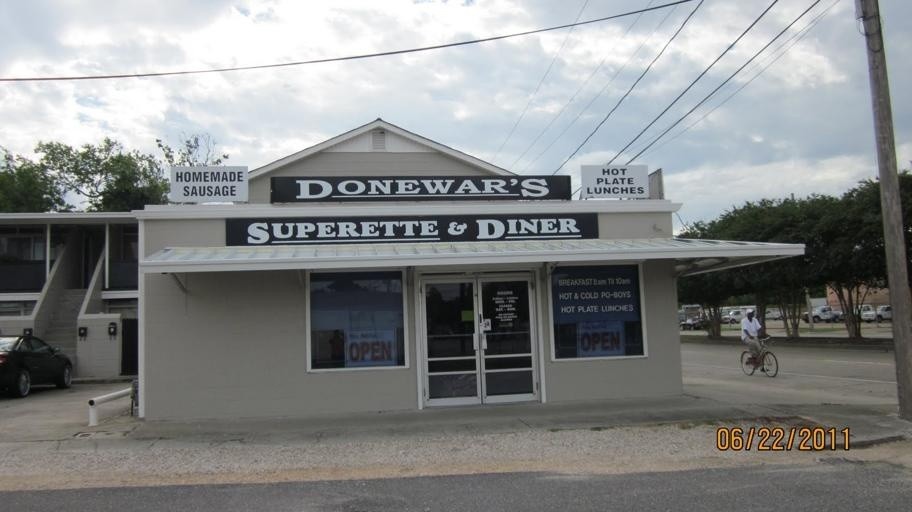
[805,306,841,323]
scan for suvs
[839,304,875,323]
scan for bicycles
[740,335,778,379]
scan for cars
[0,333,73,398]
[764,307,788,320]
[680,314,708,331]
[722,309,746,323]
[877,305,891,322]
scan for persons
[741,309,769,372]
